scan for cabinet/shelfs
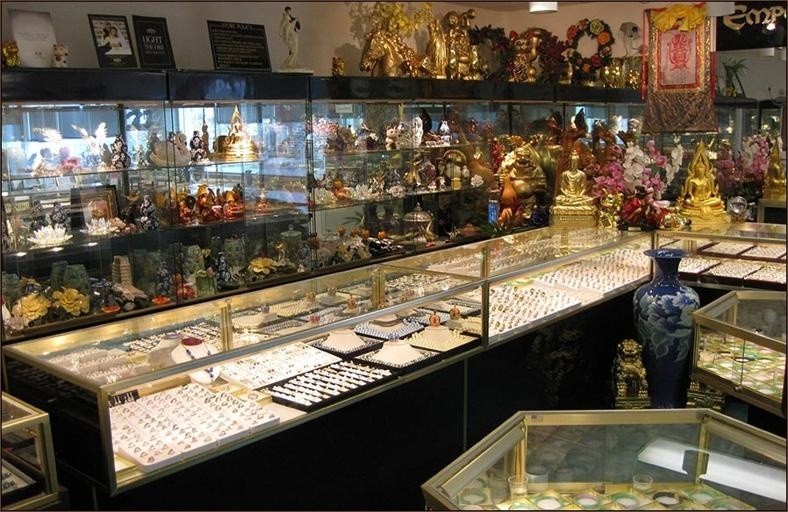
[0,67,786,511]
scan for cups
[632,474,653,497]
[509,476,528,499]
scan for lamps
[528,2,558,14]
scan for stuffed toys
[384,121,402,152]
[48,41,68,69]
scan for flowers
[565,19,615,72]
[468,24,516,81]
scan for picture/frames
[648,9,710,95]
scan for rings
[46,218,662,473]
[662,223,785,310]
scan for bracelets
[696,323,784,399]
[456,469,756,511]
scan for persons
[99,25,130,54]
[284,6,301,57]
[552,148,595,207]
[684,162,718,206]
[277,6,300,67]
[98,20,112,45]
[443,7,477,81]
[500,143,545,220]
